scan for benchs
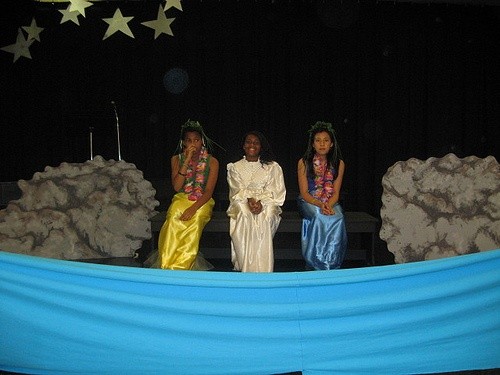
[138,179,379,266]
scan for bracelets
[177,171,188,176]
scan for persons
[297,121,348,271]
[227,131,286,273]
[153,119,220,271]
